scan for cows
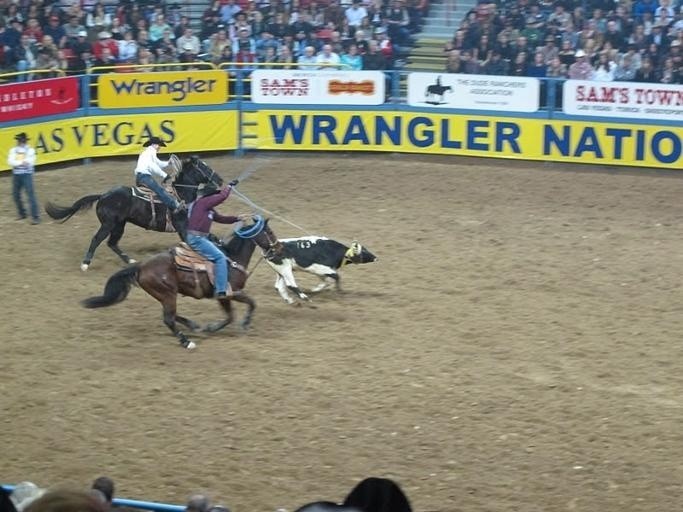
[260,235,378,306]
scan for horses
[81,216,285,351]
[44,153,225,273]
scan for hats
[14,133,30,141]
[195,185,221,195]
[183,43,193,50]
[373,27,386,34]
[9,477,113,512]
[295,477,411,512]
[97,31,112,38]
[143,137,167,147]
[187,494,231,512]
[78,30,88,36]
[574,49,589,58]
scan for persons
[113,506,149,512]
[187,494,229,511]
[5,132,40,226]
[443,0,683,85]
[1,476,114,511]
[295,477,413,511]
[1,0,431,106]
[186,179,243,303]
[133,136,186,215]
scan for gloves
[230,180,238,186]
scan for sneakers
[217,294,236,302]
[31,218,41,225]
[173,200,186,214]
